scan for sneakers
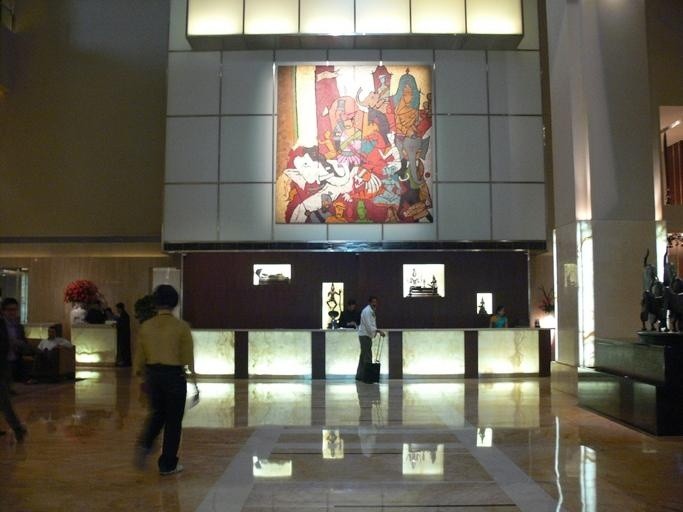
[15,428,26,441]
[159,464,183,476]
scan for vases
[69,302,89,328]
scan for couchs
[22,339,76,384]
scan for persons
[488,305,508,328]
[0,296,37,436]
[354,382,378,462]
[131,283,197,476]
[26,327,73,385]
[106,302,131,367]
[337,299,360,330]
[354,296,385,381]
[0,379,27,444]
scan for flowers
[63,278,107,307]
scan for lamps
[411,0,466,51]
[300,0,355,51]
[355,1,411,52]
[244,1,300,52]
[466,1,523,51]
[182,0,244,52]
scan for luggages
[362,334,385,384]
